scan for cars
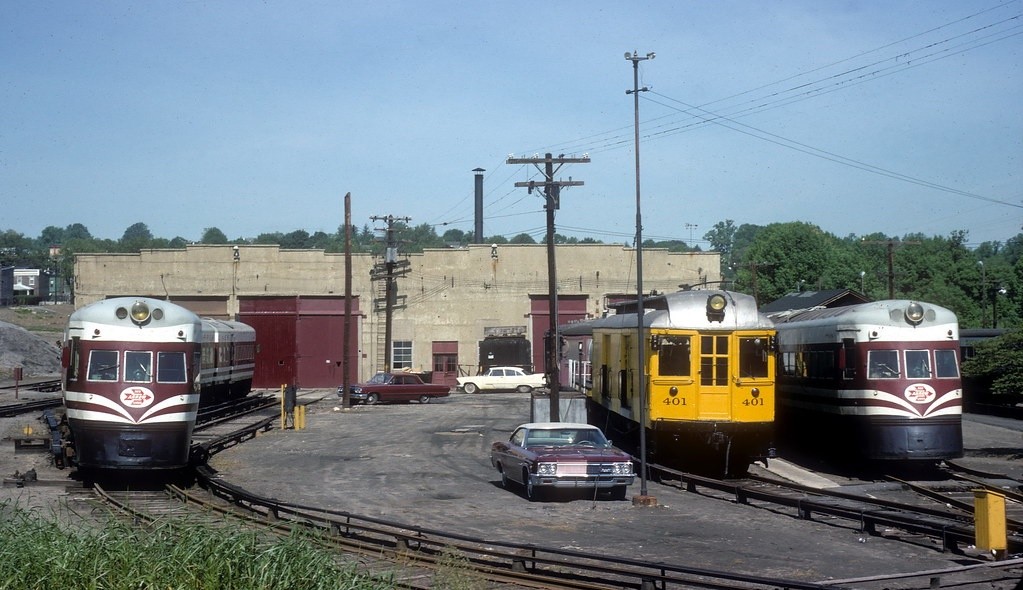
[335,372,452,404]
[490,422,637,502]
[453,367,551,393]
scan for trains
[763,298,966,467]
[590,289,780,483]
[540,319,593,392]
[57,295,259,485]
